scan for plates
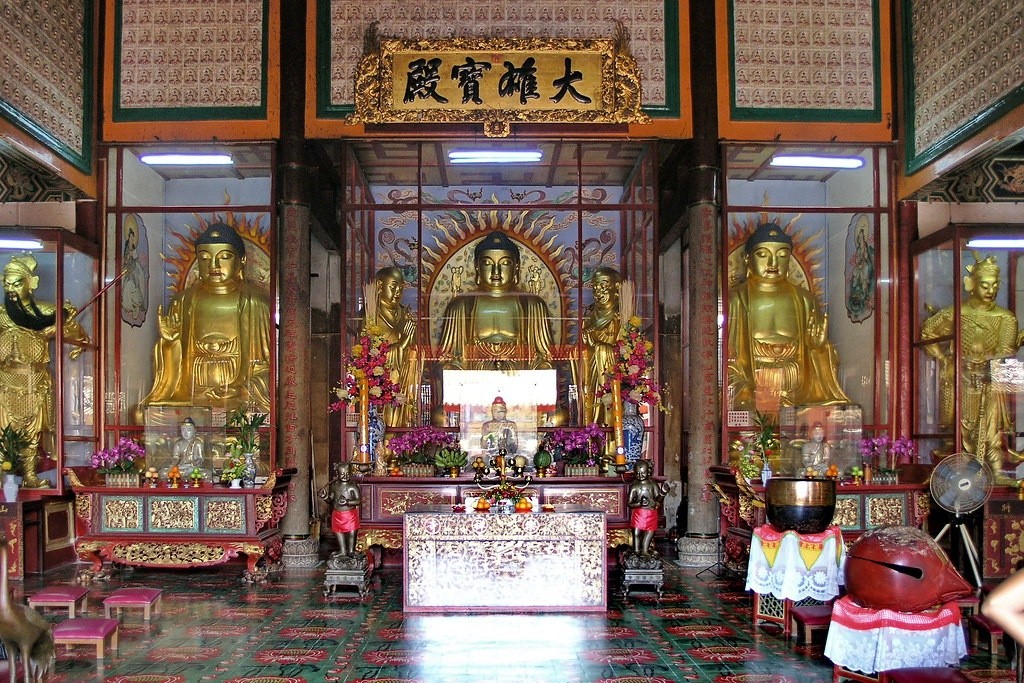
[515,507,533,510]
[452,507,465,510]
[542,507,555,510]
[473,507,489,510]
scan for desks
[824,596,966,683]
[745,526,845,629]
[402,503,608,614]
[60,464,298,582]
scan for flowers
[546,425,606,465]
[747,408,781,465]
[221,436,241,482]
[860,435,910,469]
[1,463,16,474]
[91,436,146,473]
[328,317,410,411]
[387,426,453,463]
[596,317,672,418]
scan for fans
[925,453,999,587]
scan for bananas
[436,449,468,467]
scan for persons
[318,463,362,558]
[473,397,526,467]
[129,221,270,420]
[157,417,207,477]
[627,460,669,555]
[727,223,849,410]
[575,266,620,462]
[797,422,842,477]
[921,249,1024,489]
[359,268,418,455]
[0,256,91,486]
[426,231,566,427]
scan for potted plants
[220,401,272,485]
[0,421,34,485]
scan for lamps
[448,145,545,166]
[965,234,1024,254]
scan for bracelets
[659,493,665,497]
[322,496,328,500]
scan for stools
[103,587,164,622]
[29,583,91,619]
[52,619,122,658]
[942,595,986,645]
[972,614,1010,655]
[789,603,834,640]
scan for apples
[168,466,181,478]
[515,498,532,508]
[391,467,403,475]
[190,468,202,478]
[805,466,818,476]
[826,464,838,476]
[477,498,491,508]
[851,466,863,475]
[472,457,486,467]
[145,467,159,477]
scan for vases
[869,471,899,487]
[760,463,773,487]
[103,469,140,486]
[354,402,384,463]
[228,475,243,489]
[391,460,436,477]
[4,474,20,502]
[562,460,605,475]
[616,397,647,471]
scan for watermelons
[534,450,551,466]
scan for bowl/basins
[766,480,835,534]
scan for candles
[610,380,626,463]
[358,378,370,461]
